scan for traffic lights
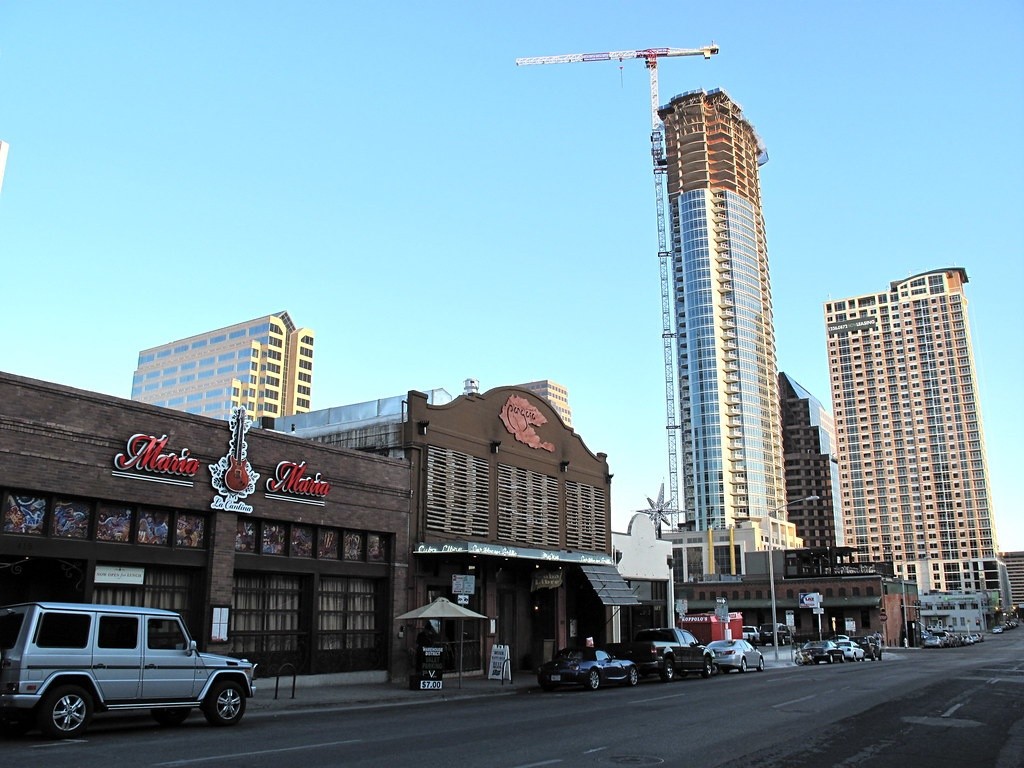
[976,620,980,625]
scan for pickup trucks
[598,628,715,682]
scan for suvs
[760,623,796,646]
[0,602,258,740]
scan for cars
[742,626,760,644]
[707,639,765,673]
[921,624,985,649]
[800,633,882,665]
[992,615,1019,634]
[536,647,639,691]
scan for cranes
[516,40,720,534]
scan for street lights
[769,496,819,662]
[901,556,930,639]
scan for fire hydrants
[903,638,908,649]
